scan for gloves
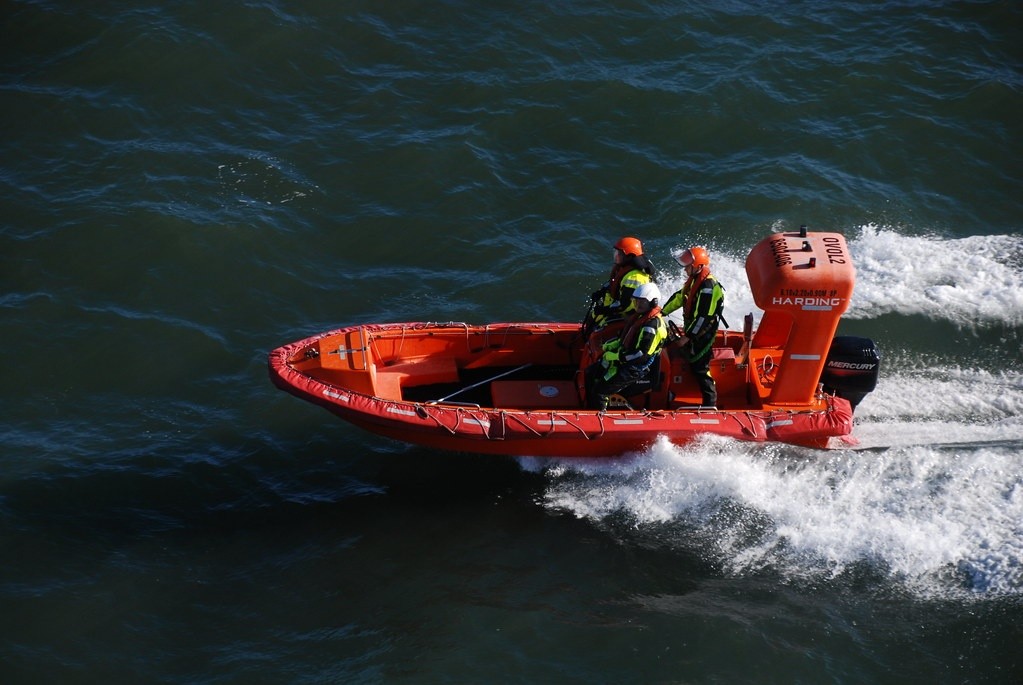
[603,351,619,362]
[590,287,607,301]
[602,340,619,350]
[594,304,610,316]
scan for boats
[268,223,881,456]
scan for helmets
[614,237,642,257]
[633,283,660,306]
[680,248,708,268]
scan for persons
[581,237,652,343]
[585,282,666,409]
[660,247,723,406]
